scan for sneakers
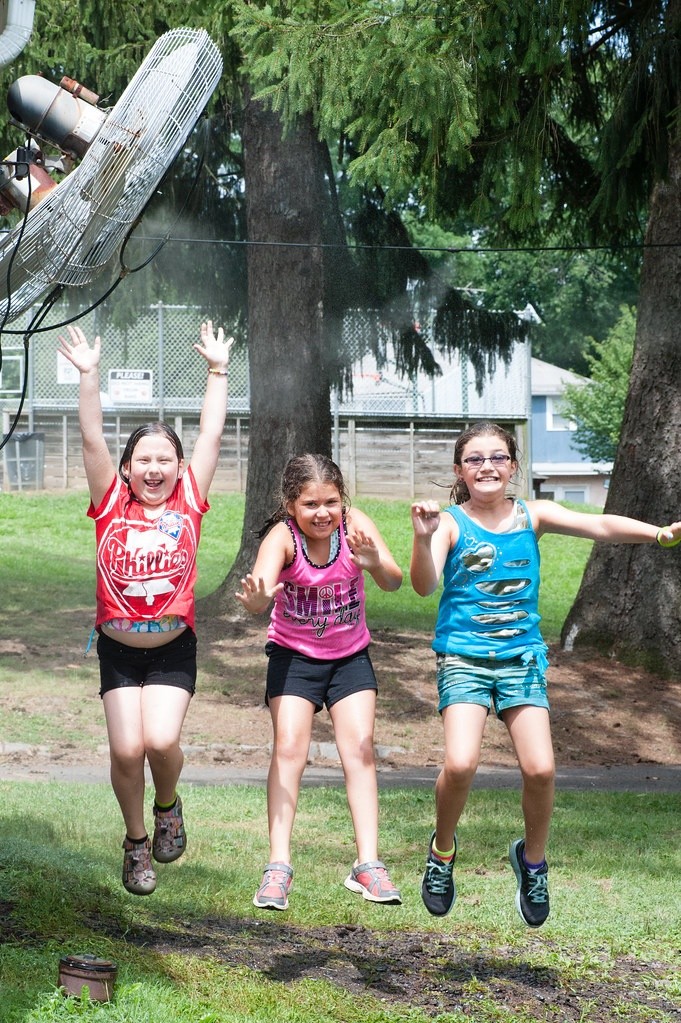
[509,838,549,928]
[343,858,402,905]
[253,861,294,911]
[421,829,458,917]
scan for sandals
[122,837,156,895]
[152,793,186,863]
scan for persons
[58,321,234,895]
[235,453,404,912]
[409,421,681,928]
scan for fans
[0,26,225,338]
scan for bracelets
[208,368,229,377]
[655,526,681,548]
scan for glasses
[460,455,511,466]
